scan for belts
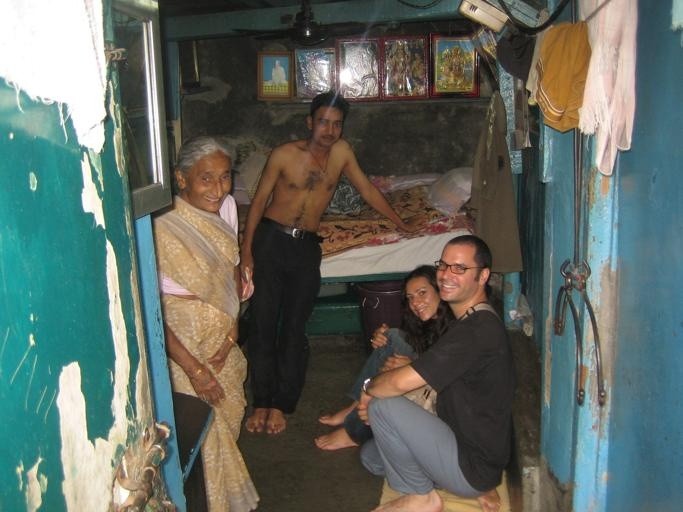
[260,216,316,239]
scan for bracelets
[191,364,203,381]
[226,333,237,347]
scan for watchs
[363,375,373,392]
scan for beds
[230,146,521,328]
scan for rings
[371,337,374,343]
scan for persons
[359,234,513,511]
[313,262,457,453]
[239,93,429,435]
[153,135,260,512]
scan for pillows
[429,166,487,218]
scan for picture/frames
[255,32,482,102]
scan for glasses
[433,261,484,274]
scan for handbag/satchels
[403,384,440,418]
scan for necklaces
[303,139,332,176]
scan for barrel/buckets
[357,280,407,360]
